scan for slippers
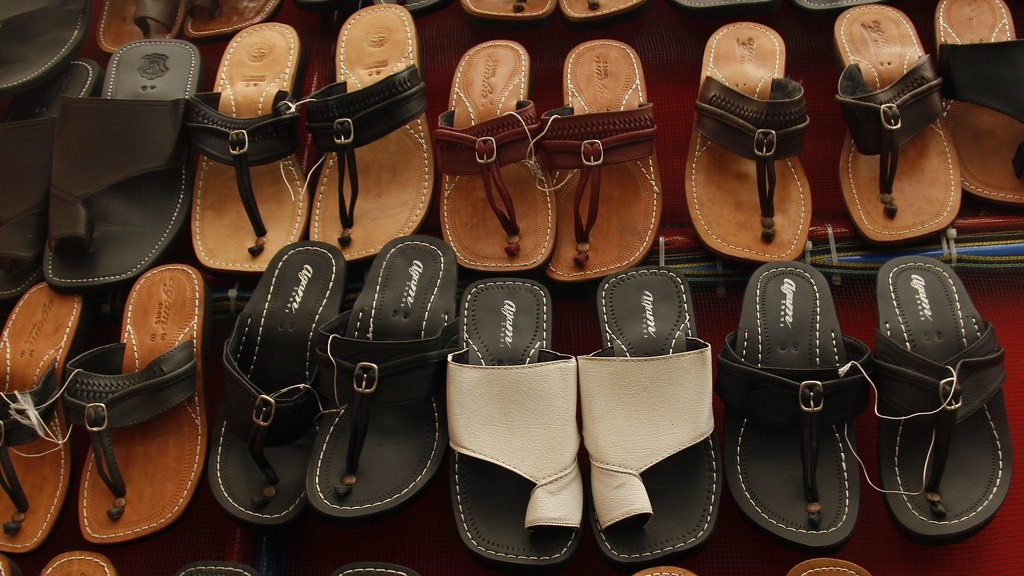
[0,0,1024,576]
